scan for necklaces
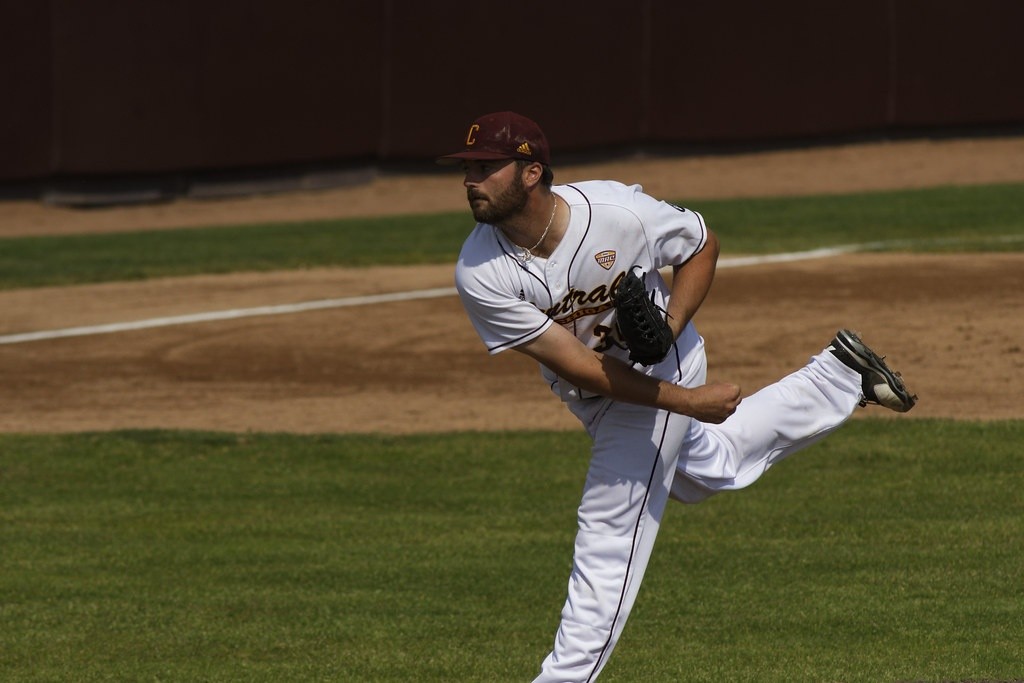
[498,191,557,265]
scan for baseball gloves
[607,263,675,368]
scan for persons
[436,110,917,683]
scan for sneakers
[826,328,918,413]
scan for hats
[434,111,551,167]
